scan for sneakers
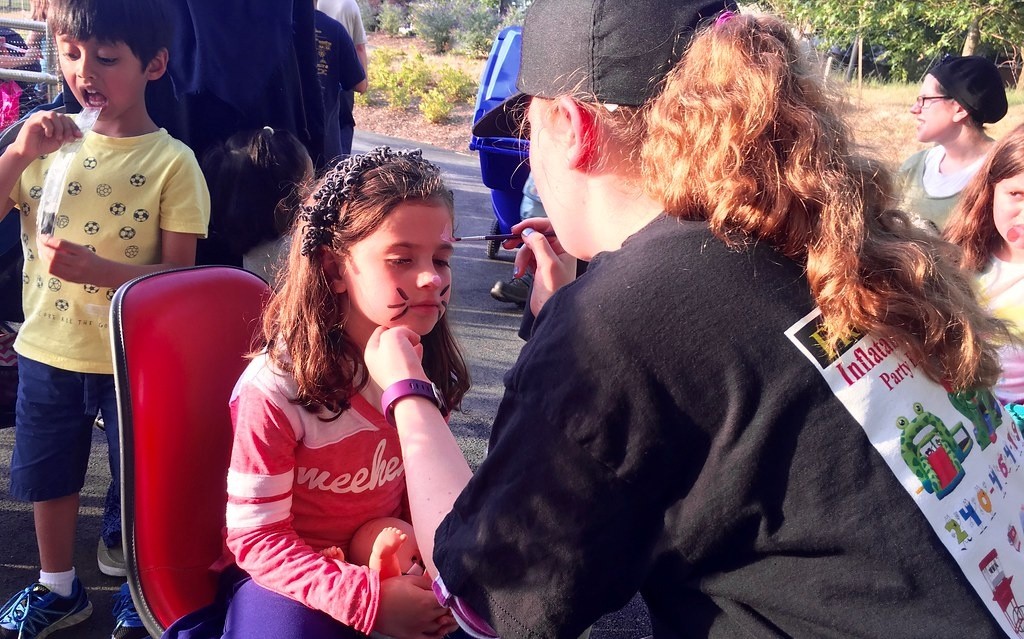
[0,576,93,639]
[109,582,152,639]
[96,534,126,577]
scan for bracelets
[382,380,447,426]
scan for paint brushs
[449,230,555,242]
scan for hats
[471,0,740,137]
[929,56,1008,125]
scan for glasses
[916,96,952,107]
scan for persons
[940,124,1024,406]
[317,0,368,154]
[313,0,366,157]
[898,56,1008,234]
[364,0,1024,639]
[491,172,546,310]
[97,0,320,577]
[319,517,421,578]
[208,146,469,639]
[0,0,211,639]
[202,127,314,282]
[0,0,56,99]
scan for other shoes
[489,278,530,305]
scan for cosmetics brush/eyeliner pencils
[455,232,556,241]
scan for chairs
[107,263,280,639]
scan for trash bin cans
[470,24,531,258]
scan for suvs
[1,26,48,122]
